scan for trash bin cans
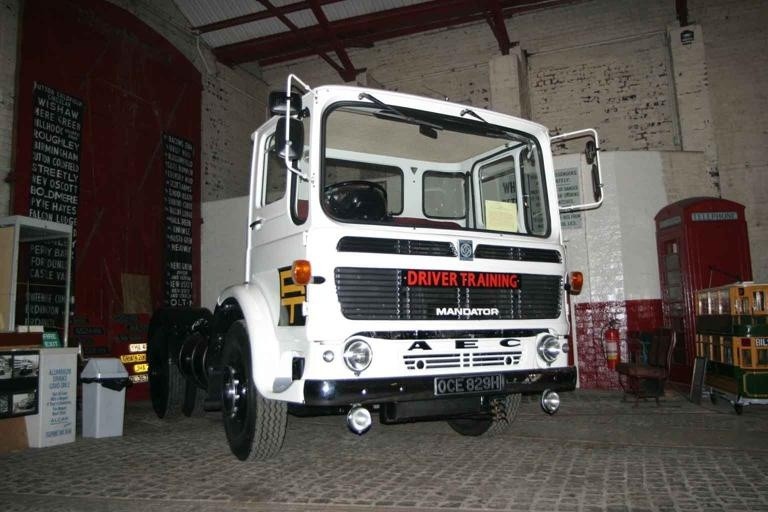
[80,358,129,439]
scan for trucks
[145,69,608,462]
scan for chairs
[616,325,677,409]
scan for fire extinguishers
[600,318,621,369]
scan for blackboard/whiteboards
[162,133,195,310]
[24,82,82,332]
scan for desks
[0,345,78,454]
